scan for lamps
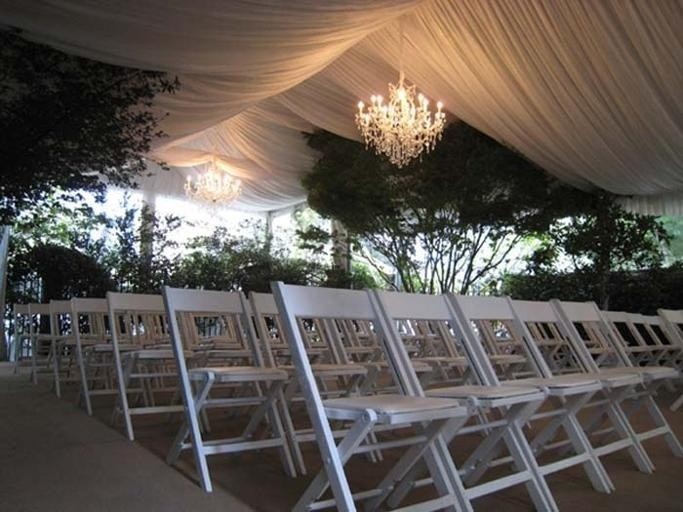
[184,127,242,213]
[354,14,446,171]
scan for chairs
[14,281,683,512]
